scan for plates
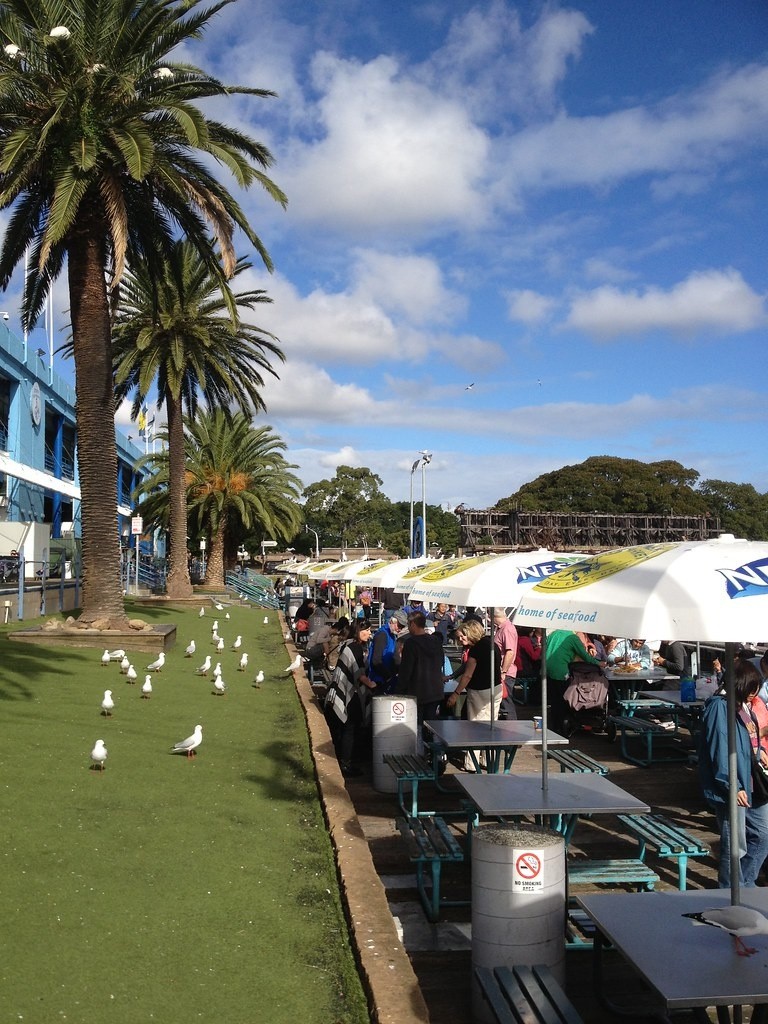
[612,670,640,674]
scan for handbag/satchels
[680,669,696,702]
[752,762,768,809]
[295,619,308,631]
[696,673,718,701]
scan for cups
[533,716,543,730]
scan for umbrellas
[307,562,349,604]
[326,558,385,618]
[350,557,441,629]
[511,533,768,908]
[273,562,312,586]
[392,554,476,594]
[408,548,594,791]
[297,562,324,602]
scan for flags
[143,415,154,443]
[139,406,148,436]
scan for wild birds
[230,636,242,652]
[193,656,212,677]
[419,450,428,455]
[464,382,474,390]
[537,379,542,386]
[215,604,230,619]
[102,649,138,685]
[264,616,269,624]
[252,670,265,686]
[239,652,249,672]
[284,634,289,641]
[239,592,248,601]
[213,663,225,695]
[199,607,204,618]
[143,652,166,673]
[170,724,203,759]
[282,654,302,672]
[90,739,107,771]
[142,675,152,699]
[184,639,196,657]
[211,620,225,653]
[101,690,114,718]
[258,589,276,602]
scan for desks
[422,719,569,774]
[573,889,768,1023]
[638,690,729,708]
[600,663,681,680]
[453,773,651,862]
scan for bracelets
[448,676,450,679]
[455,690,460,695]
[450,675,453,679]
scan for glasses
[359,618,369,627]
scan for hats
[393,610,409,626]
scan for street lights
[420,449,433,559]
[411,460,420,558]
[306,524,320,558]
[202,537,207,579]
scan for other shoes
[340,760,363,777]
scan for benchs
[477,963,585,1024]
[423,741,610,774]
[610,699,705,767]
[382,753,436,815]
[395,815,471,923]
[513,675,543,705]
[458,797,711,949]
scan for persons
[139,550,143,561]
[275,578,281,594]
[285,579,768,889]
[4,550,20,582]
